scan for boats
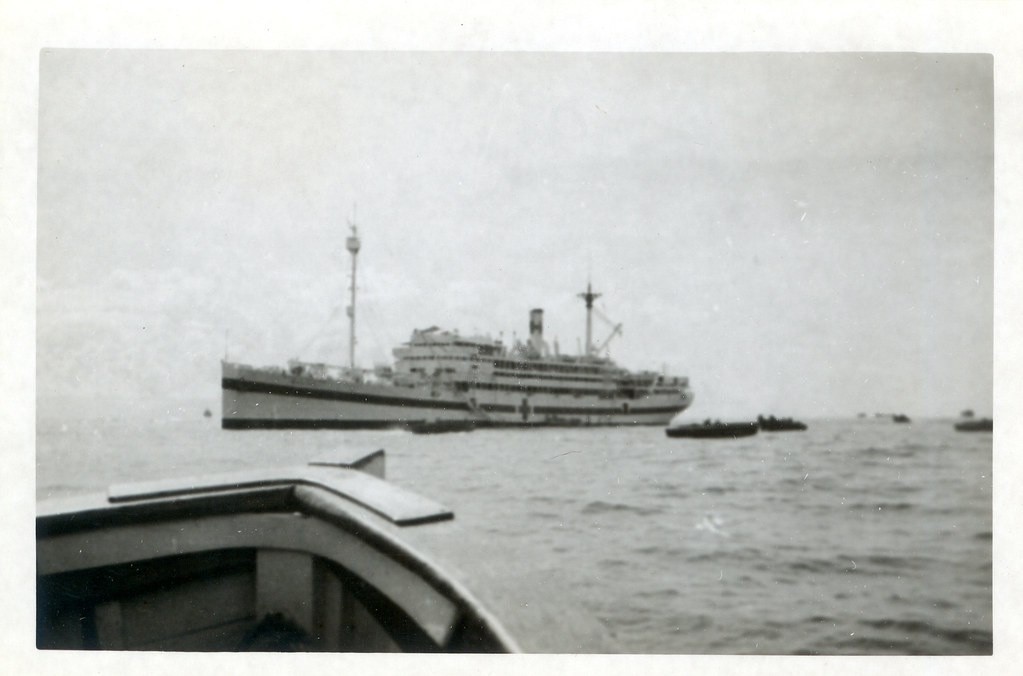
[756,414,811,431]
[218,197,695,432]
[665,423,758,438]
